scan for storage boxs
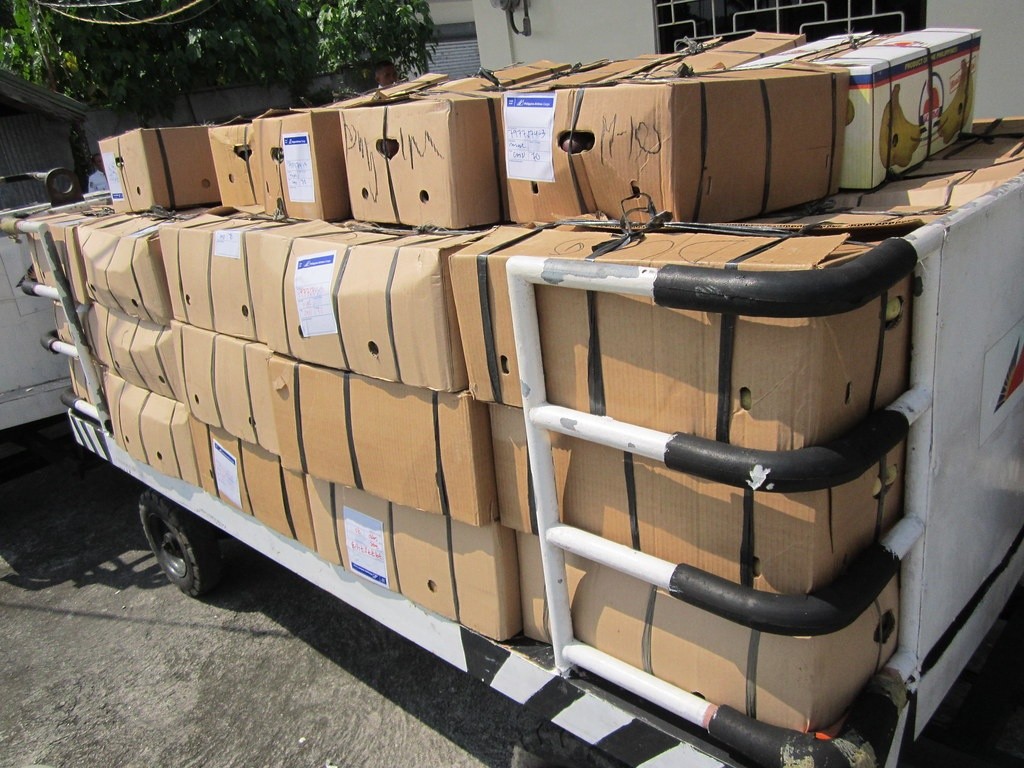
[26,23,1024,737]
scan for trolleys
[0,169,1024,768]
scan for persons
[87,154,109,193]
[365,60,397,90]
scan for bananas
[879,59,975,168]
